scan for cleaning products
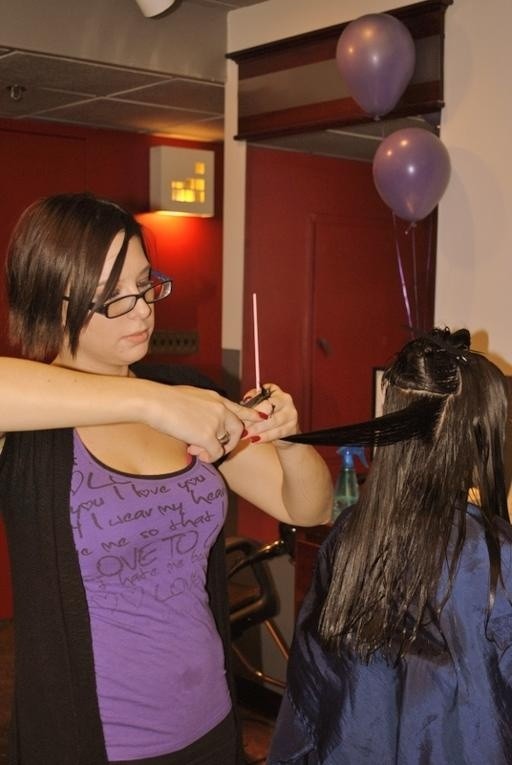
[331,446,368,526]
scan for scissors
[243,390,267,409]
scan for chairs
[224,535,290,765]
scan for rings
[218,431,230,444]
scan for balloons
[336,12,417,119]
[372,127,452,227]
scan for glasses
[63,268,174,319]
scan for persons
[270,325,510,764]
[0,195,335,764]
